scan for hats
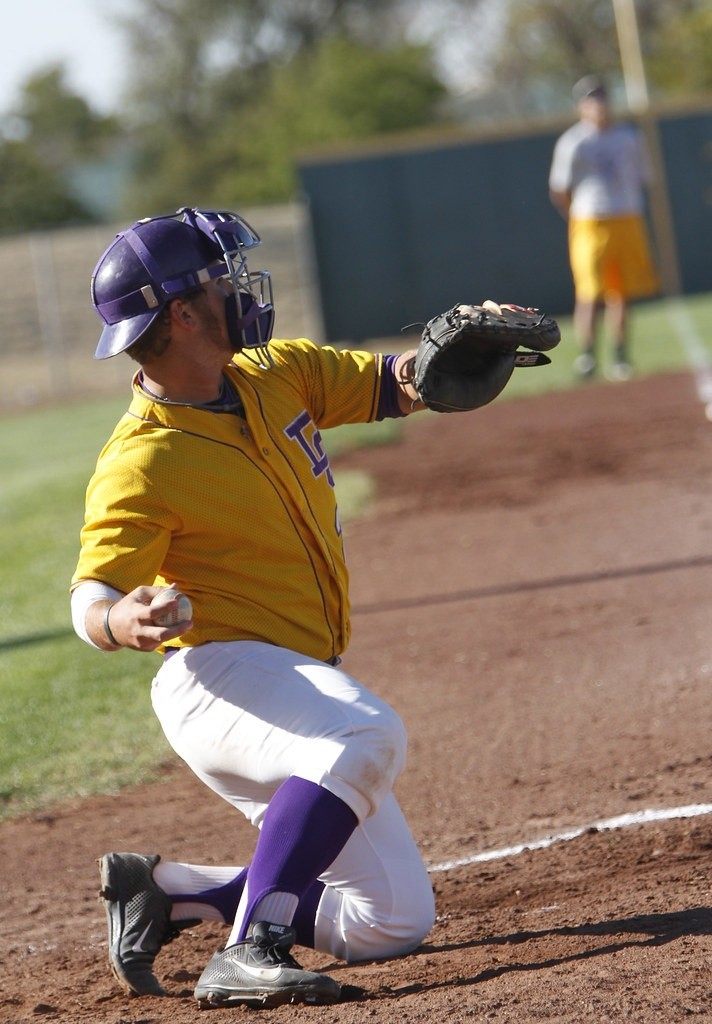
[572,74,609,101]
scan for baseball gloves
[413,299,562,413]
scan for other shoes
[605,359,635,382]
[574,353,595,379]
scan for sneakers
[95,852,203,997]
[193,921,340,1009]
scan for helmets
[90,216,221,360]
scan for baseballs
[151,589,192,633]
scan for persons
[67,207,562,1009]
[549,72,660,381]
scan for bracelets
[105,603,121,646]
